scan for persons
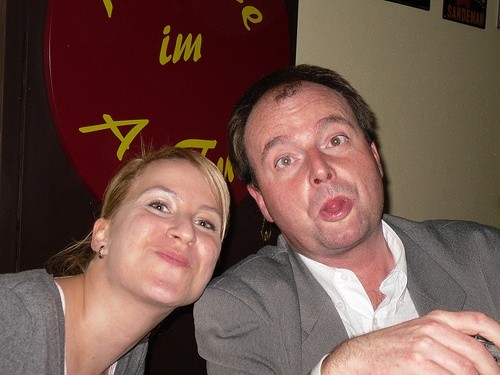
[0,144,232,375]
[193,65,500,375]
